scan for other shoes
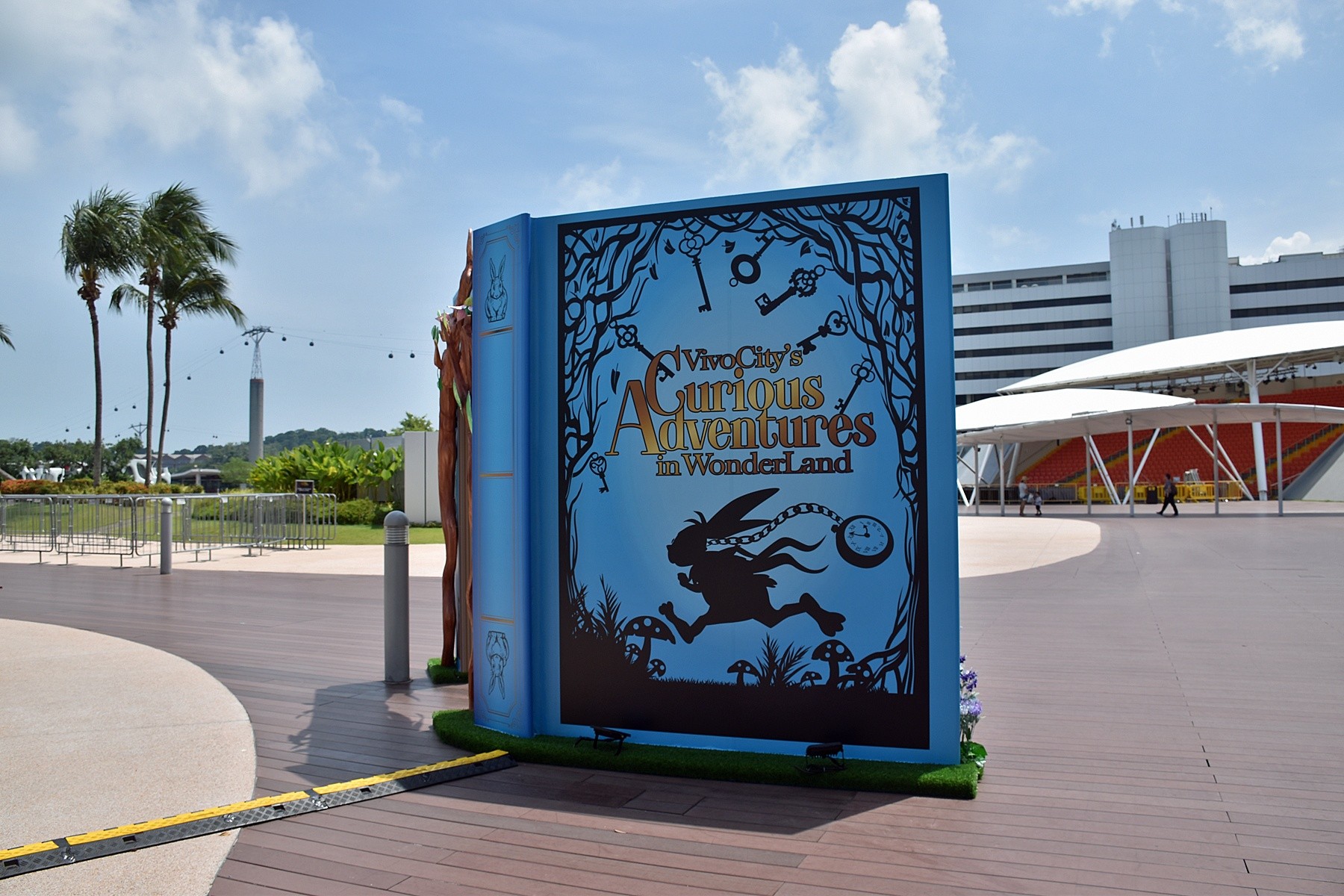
[1173,513,1178,515]
[1157,512,1163,515]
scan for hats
[1020,475,1030,481]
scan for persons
[1034,491,1042,516]
[1019,476,1030,517]
[1156,474,1179,517]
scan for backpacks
[1166,482,1178,497]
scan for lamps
[1158,364,1317,396]
